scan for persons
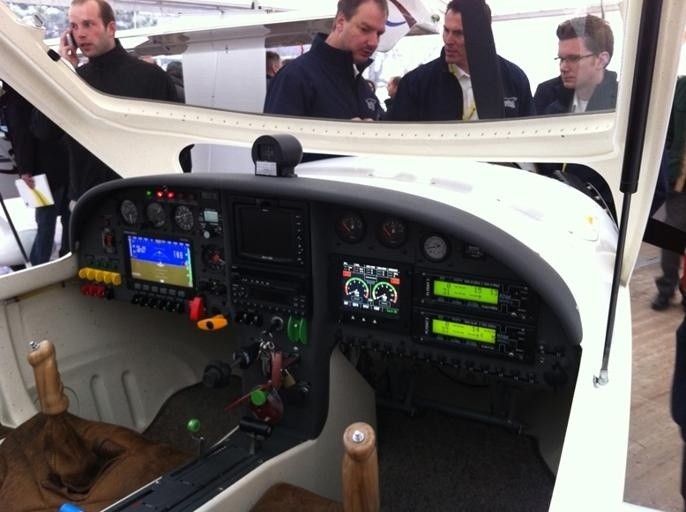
[535,15,617,174]
[384,76,401,108]
[138,56,156,64]
[367,80,376,94]
[265,51,282,85]
[390,0,533,169]
[264,0,389,163]
[651,77,686,310]
[167,61,183,103]
[57,0,179,201]
[2,82,70,265]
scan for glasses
[553,53,597,63]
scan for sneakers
[652,289,675,311]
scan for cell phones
[66,33,77,49]
